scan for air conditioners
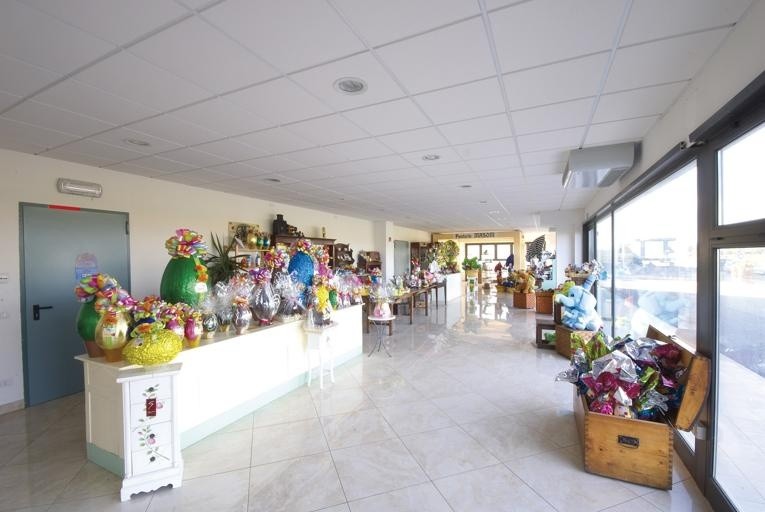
[57,178,102,198]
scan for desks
[362,278,447,337]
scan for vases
[74,229,364,365]
[272,213,288,234]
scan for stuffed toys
[553,274,606,334]
[515,269,536,295]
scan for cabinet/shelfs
[410,241,440,274]
[357,249,382,274]
[536,275,596,349]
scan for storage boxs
[573,323,715,492]
[513,291,536,310]
[535,292,555,314]
[555,325,595,360]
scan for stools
[367,314,396,360]
[303,321,339,390]
